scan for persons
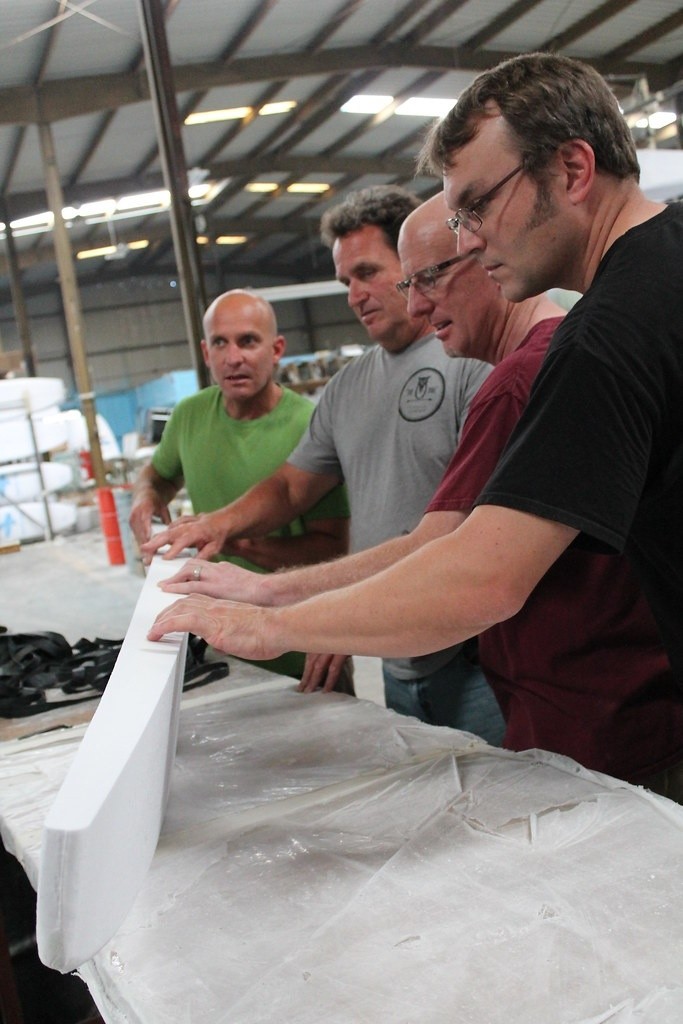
[139,183,510,753]
[129,287,353,695]
[149,51,683,661]
[157,191,683,805]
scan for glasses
[446,162,524,235]
[396,257,462,301]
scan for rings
[192,567,202,582]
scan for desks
[0,538,683,1024]
